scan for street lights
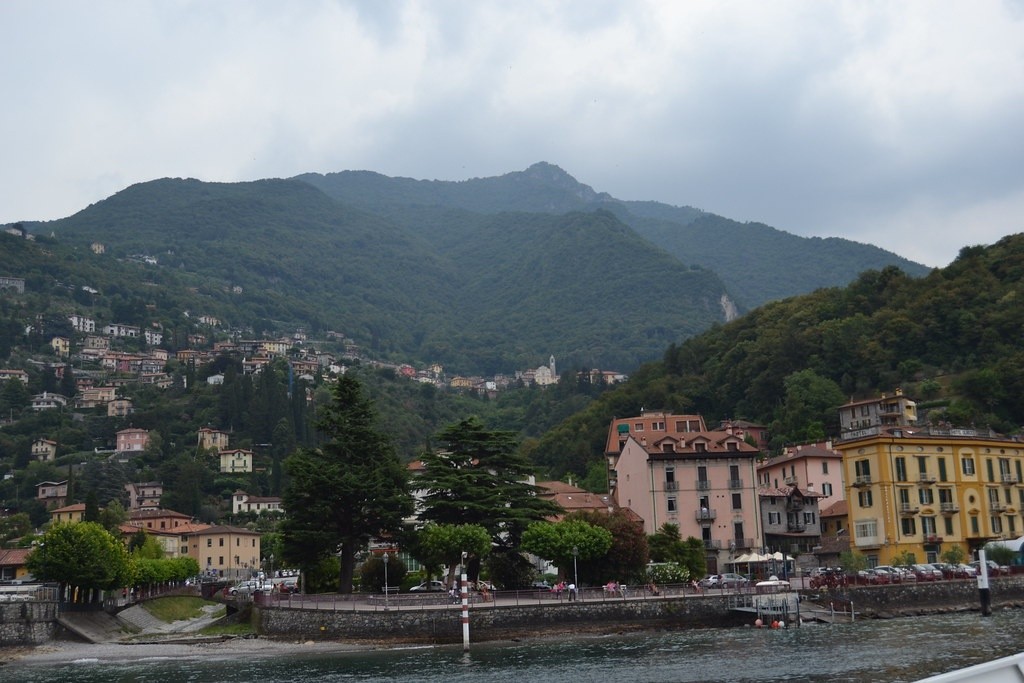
[460,551,468,567]
[382,551,391,613]
[731,542,738,593]
[570,546,580,603]
[763,545,771,578]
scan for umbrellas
[725,551,797,581]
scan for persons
[607,582,625,598]
[543,579,576,602]
[648,581,658,595]
[272,584,278,592]
[123,588,127,602]
[448,584,491,604]
[691,577,702,594]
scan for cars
[257,571,265,582]
[702,574,751,589]
[409,581,446,593]
[483,581,496,591]
[808,559,1013,588]
[260,580,275,591]
[279,580,297,592]
[229,580,263,596]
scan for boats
[727,604,798,627]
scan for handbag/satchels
[551,589,556,592]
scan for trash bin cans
[484,592,493,603]
[1000,565,1010,576]
[568,589,578,601]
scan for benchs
[602,584,627,598]
[553,584,579,598]
[382,586,400,595]
